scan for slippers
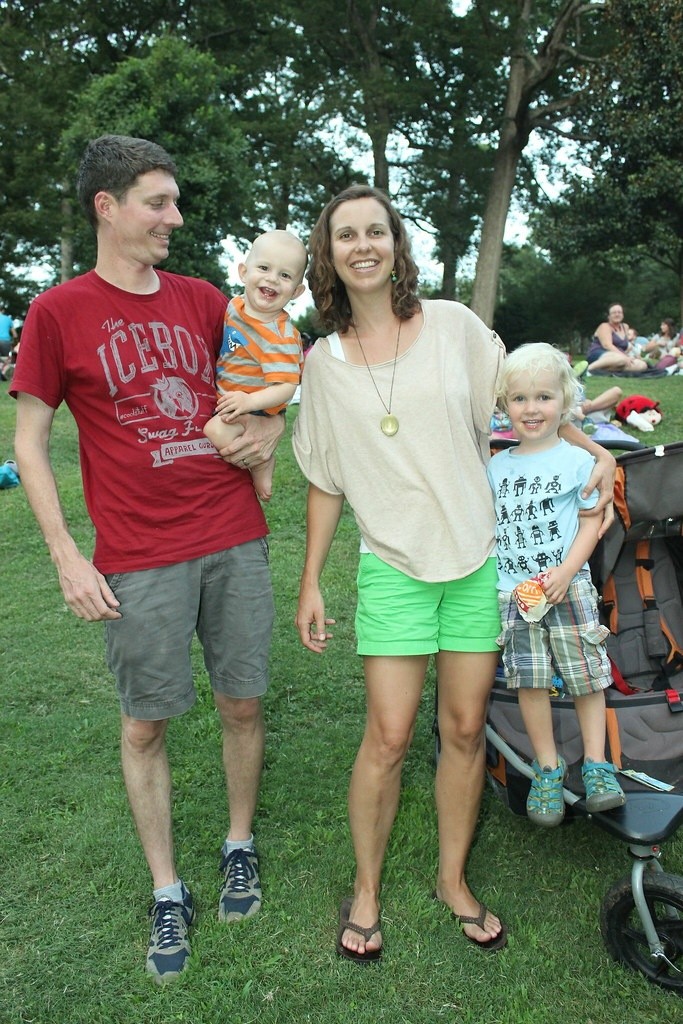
[432,886,508,953]
[337,896,383,963]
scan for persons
[293,183,628,964]
[8,133,306,987]
[0,304,20,382]
[572,302,683,378]
[572,382,624,435]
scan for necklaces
[349,307,404,437]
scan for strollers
[432,439,682,994]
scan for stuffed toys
[615,395,663,432]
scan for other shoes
[581,756,627,813]
[574,360,592,379]
[526,754,569,828]
[581,416,599,435]
[665,363,679,376]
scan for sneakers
[145,880,197,993]
[217,840,262,925]
